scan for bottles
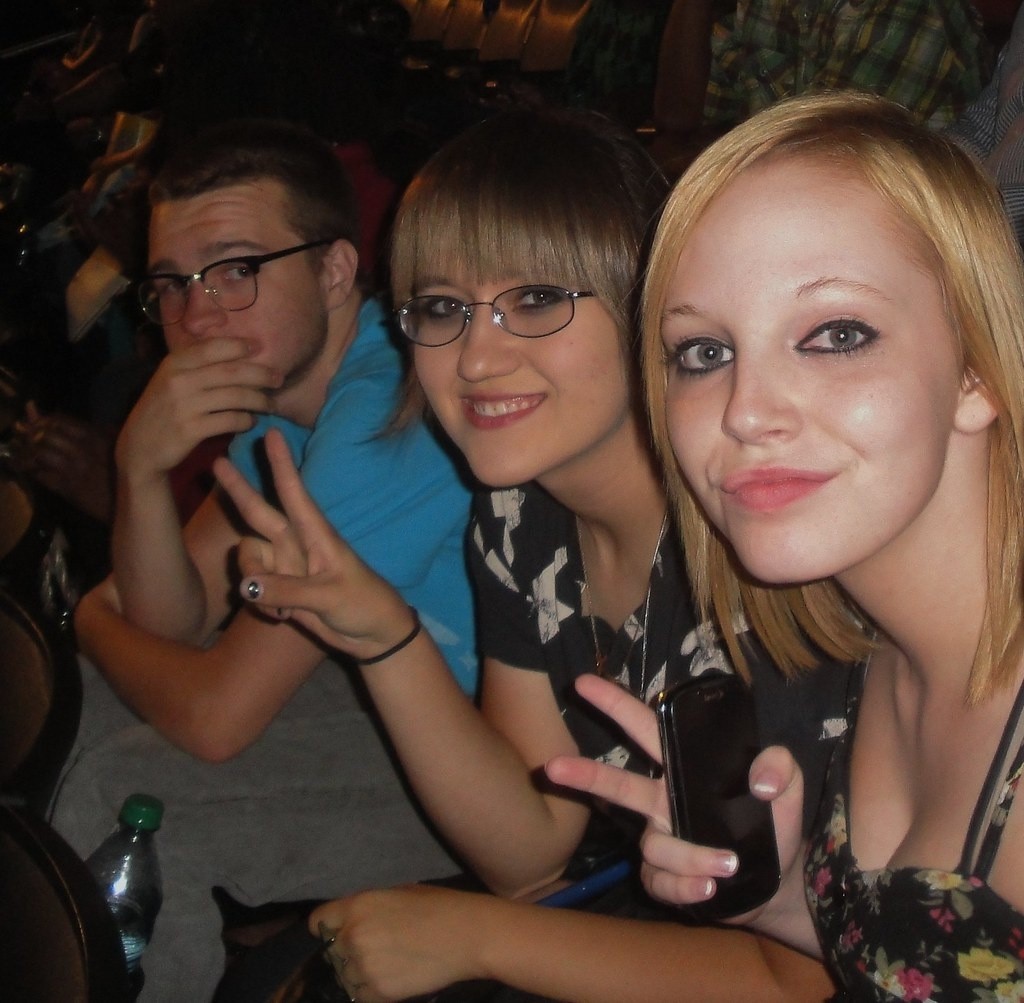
[83,793,164,972]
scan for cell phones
[657,673,781,923]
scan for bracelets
[354,606,421,665]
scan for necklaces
[563,485,671,705]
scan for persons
[543,87,1024,1003]
[45,139,480,1003]
[0,0,948,475]
[209,109,837,1003]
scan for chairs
[1,376,171,1003]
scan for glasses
[138,237,334,326]
[391,283,593,349]
